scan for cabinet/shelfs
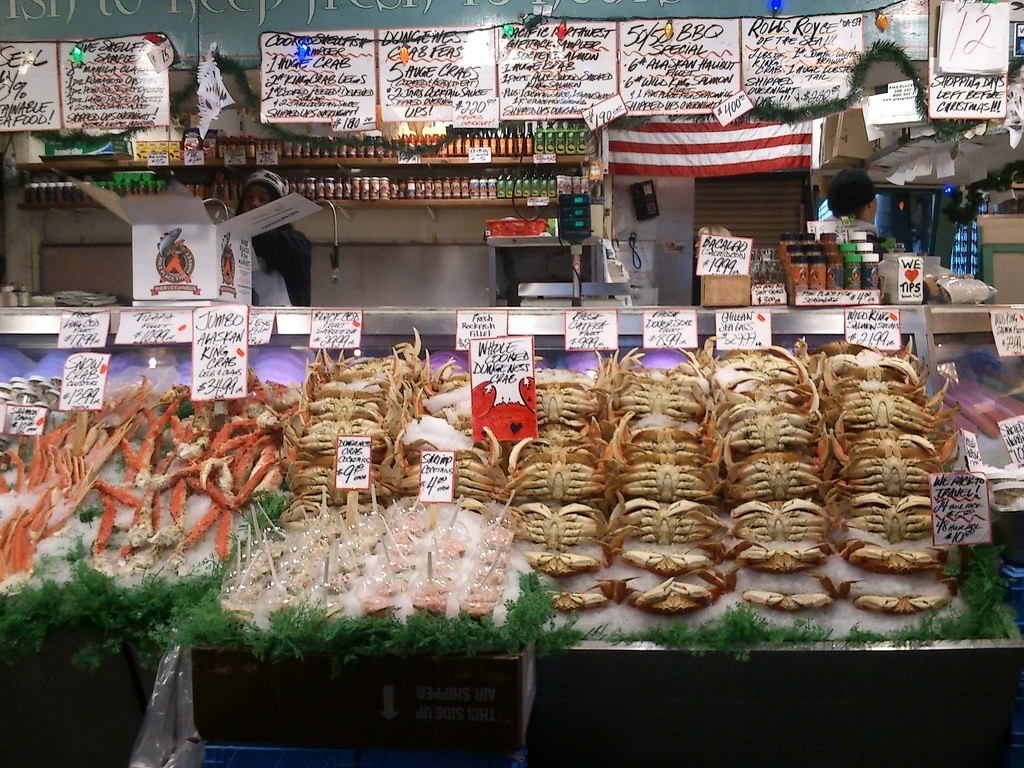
[10,156,598,223]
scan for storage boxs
[697,275,751,307]
[68,177,324,305]
[782,261,886,307]
[749,282,787,306]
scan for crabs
[604,344,743,617]
[795,335,960,613]
[279,326,610,610]
[0,369,300,583]
[704,335,839,610]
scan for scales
[518,194,630,309]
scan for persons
[692,226,732,306]
[234,169,312,307]
[828,168,941,305]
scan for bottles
[23,120,587,201]
[778,230,879,291]
[0,375,63,471]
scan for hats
[826,168,877,217]
[243,170,285,199]
[1012,171,1024,188]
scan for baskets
[485,219,548,236]
